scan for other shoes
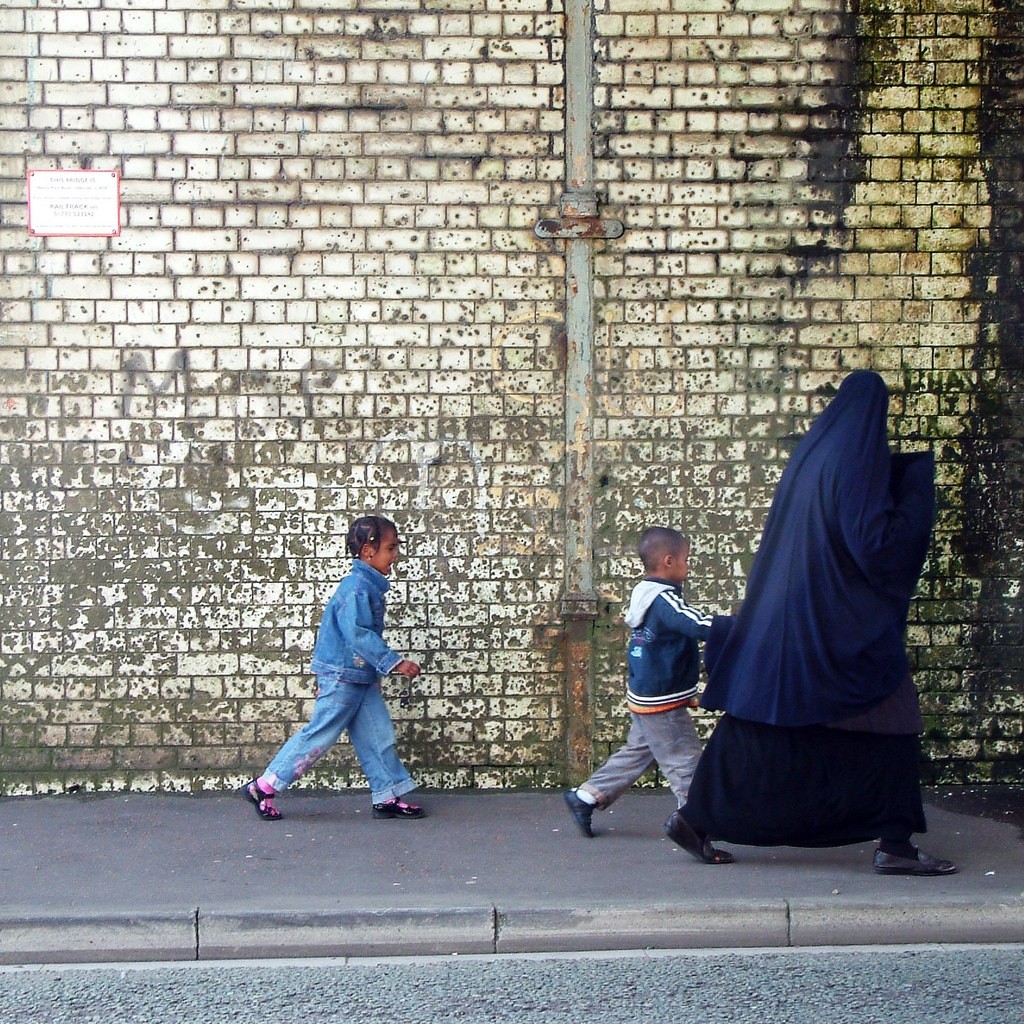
[563,787,597,838]
[372,797,425,820]
[241,776,282,821]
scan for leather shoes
[663,811,735,863]
[873,847,959,876]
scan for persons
[663,371,957,877]
[240,515,426,822]
[559,526,715,839]
